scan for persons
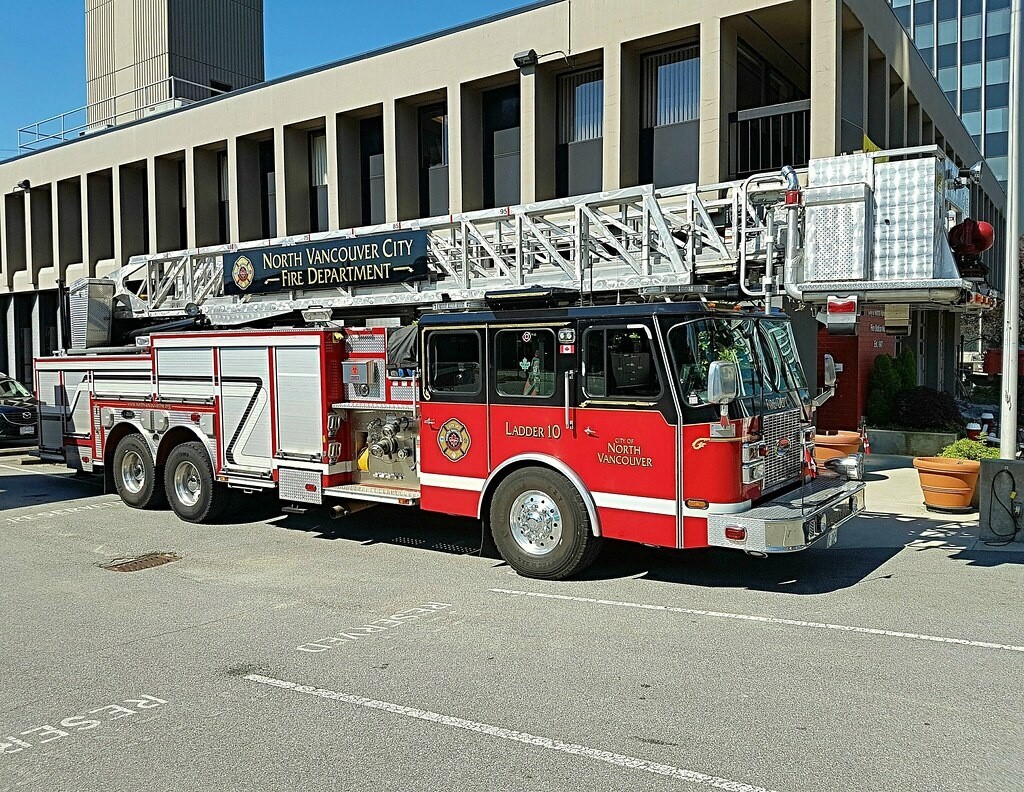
[530,350,540,377]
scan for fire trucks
[20,165,866,581]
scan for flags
[862,424,870,456]
[802,445,817,479]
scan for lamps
[513,49,538,67]
[17,179,30,190]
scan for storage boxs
[981,412,994,432]
[965,423,981,441]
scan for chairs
[611,352,650,388]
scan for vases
[815,430,862,473]
[912,457,980,509]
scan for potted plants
[935,431,1000,508]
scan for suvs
[0,372,38,445]
